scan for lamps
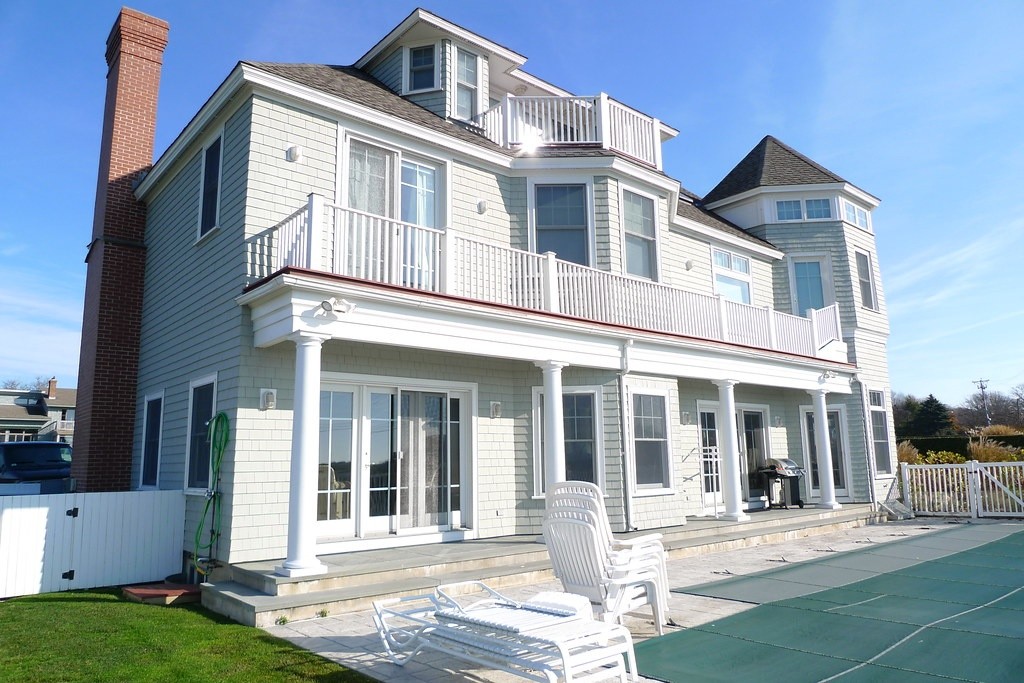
[685,259,693,270]
[321,297,357,313]
[259,389,277,411]
[682,411,692,425]
[489,400,501,419]
[476,201,488,214]
[285,146,301,162]
[824,371,837,379]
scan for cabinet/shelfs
[759,457,807,509]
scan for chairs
[370,581,638,683]
[541,480,671,636]
